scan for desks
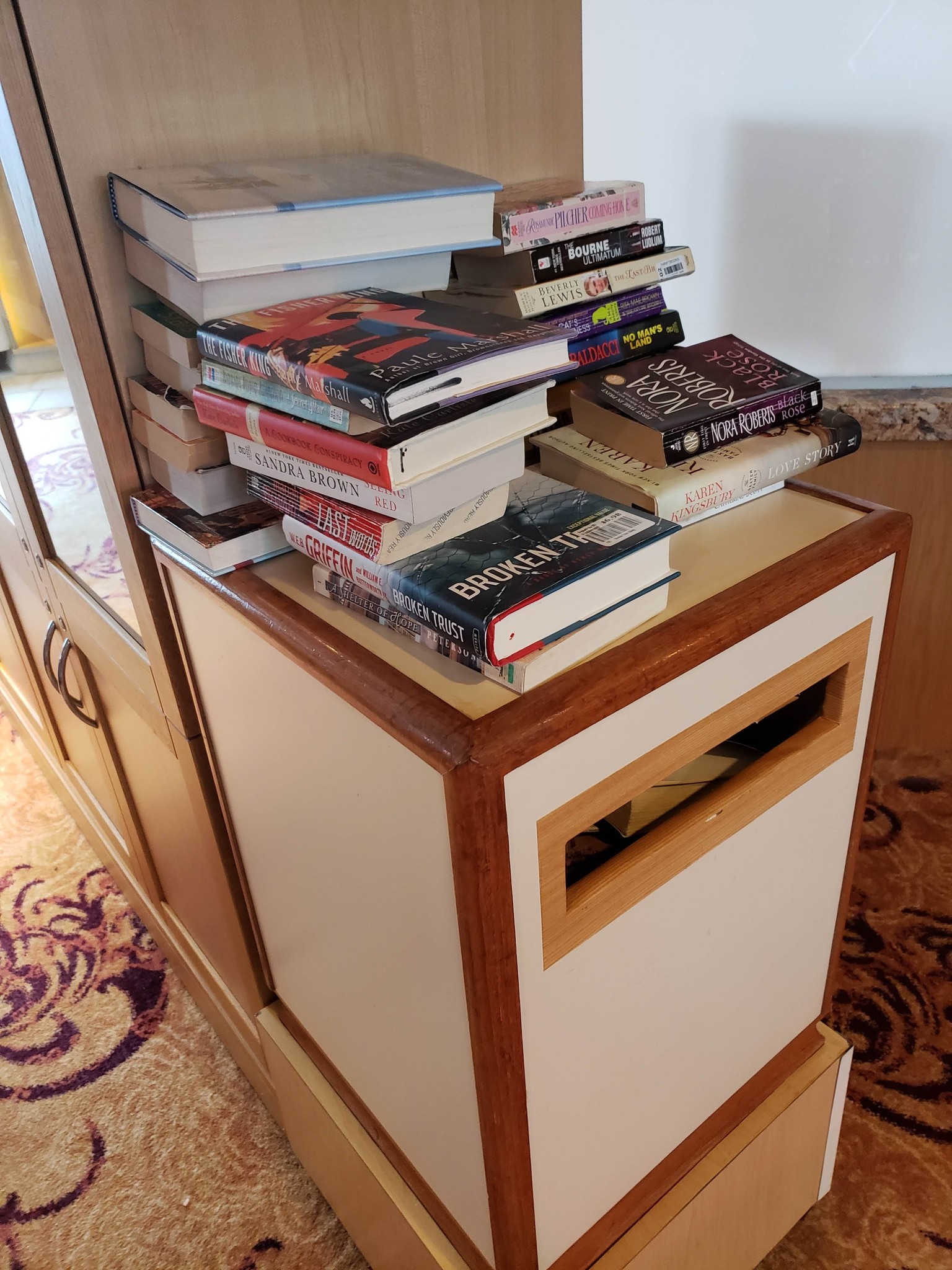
[150,458,910,1270]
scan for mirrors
[0,87,146,656]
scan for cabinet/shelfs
[0,0,582,1123]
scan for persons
[584,271,613,297]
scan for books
[311,560,669,696]
[550,310,685,382]
[108,152,505,282]
[190,377,561,493]
[454,218,665,285]
[280,466,681,667]
[127,299,261,515]
[569,333,824,469]
[223,431,526,526]
[539,285,667,343]
[423,245,695,317]
[530,407,861,527]
[201,357,384,436]
[459,180,646,259]
[131,483,295,579]
[198,287,579,427]
[677,480,786,528]
[243,470,509,565]
[121,229,454,329]
